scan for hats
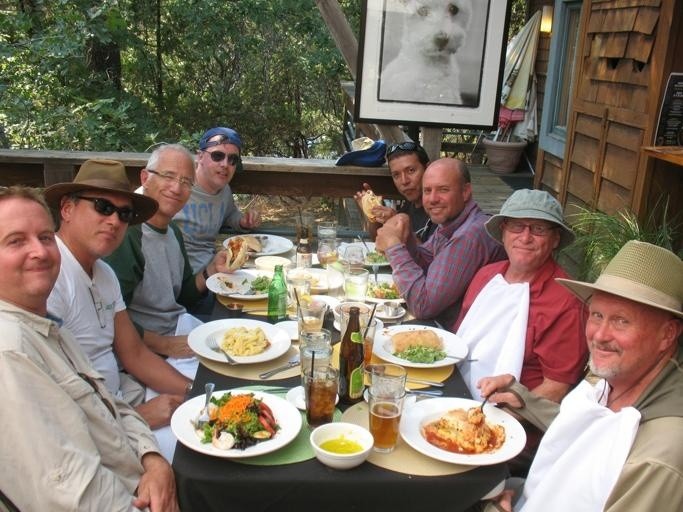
[554,238,683,321]
[40,158,160,226]
[199,127,242,159]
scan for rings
[382,213,385,217]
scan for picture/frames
[350,0,512,131]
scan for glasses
[386,141,429,158]
[77,371,117,420]
[89,283,108,329]
[483,187,578,250]
[199,149,243,166]
[144,166,199,194]
[499,221,565,237]
[65,192,138,226]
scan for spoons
[470,389,497,424]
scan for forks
[206,336,238,365]
[198,383,216,430]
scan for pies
[224,236,247,272]
[360,189,382,224]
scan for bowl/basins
[310,422,375,470]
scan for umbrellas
[468,8,542,164]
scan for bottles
[296,226,312,269]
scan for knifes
[383,372,445,388]
[258,356,300,378]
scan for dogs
[380,0,472,104]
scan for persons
[450,189,591,468]
[353,141,437,243]
[0,184,180,512]
[375,157,508,328]
[133,127,262,314]
[478,240,683,512]
[102,144,236,358]
[41,159,194,430]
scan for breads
[390,330,442,353]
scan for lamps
[537,3,553,35]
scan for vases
[482,135,527,174]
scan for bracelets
[185,379,193,400]
[203,268,209,279]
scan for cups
[367,364,407,452]
[297,300,377,430]
[317,222,336,247]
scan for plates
[399,397,527,465]
[170,389,303,458]
[285,386,340,411]
[187,318,292,364]
[372,324,469,368]
[206,233,406,341]
[363,387,417,408]
